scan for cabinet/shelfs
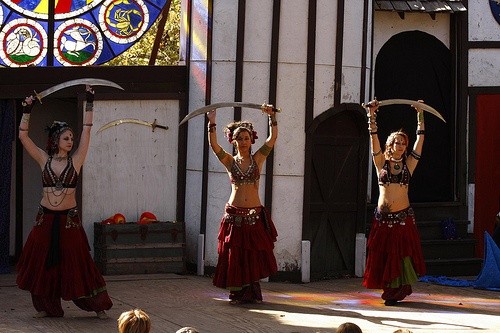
[94,222,187,274]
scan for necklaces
[389,155,403,170]
[235,155,250,165]
[52,155,67,161]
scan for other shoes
[32,310,64,318]
[381,284,413,306]
[229,294,263,304]
[96,311,107,319]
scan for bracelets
[367,110,379,135]
[82,124,93,126]
[208,123,216,132]
[19,127,29,131]
[416,130,426,135]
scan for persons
[15,78,124,319]
[176,327,199,333]
[336,322,363,333]
[178,102,281,305]
[361,98,447,306]
[393,329,414,333]
[117,308,151,333]
